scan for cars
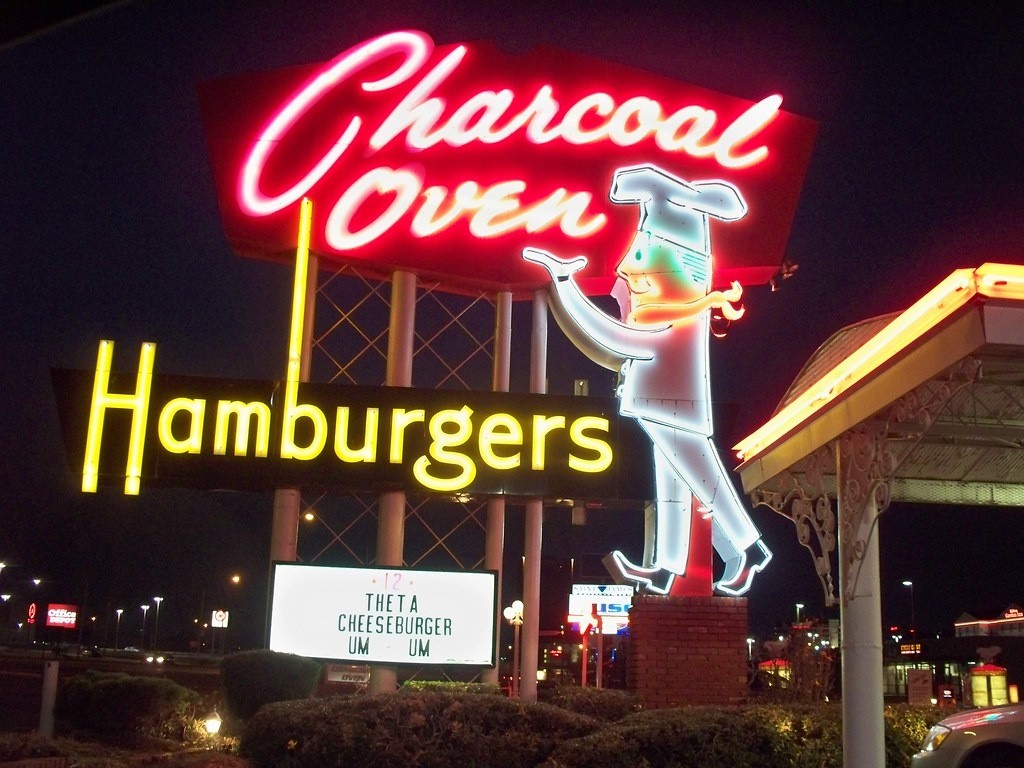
[911,703,1024,768]
[146,654,175,665]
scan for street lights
[503,600,524,701]
[153,597,164,653]
[34,578,41,646]
[141,605,150,654]
[903,581,918,669]
[747,638,756,659]
[1,592,11,646]
[113,609,123,652]
[796,603,805,624]
